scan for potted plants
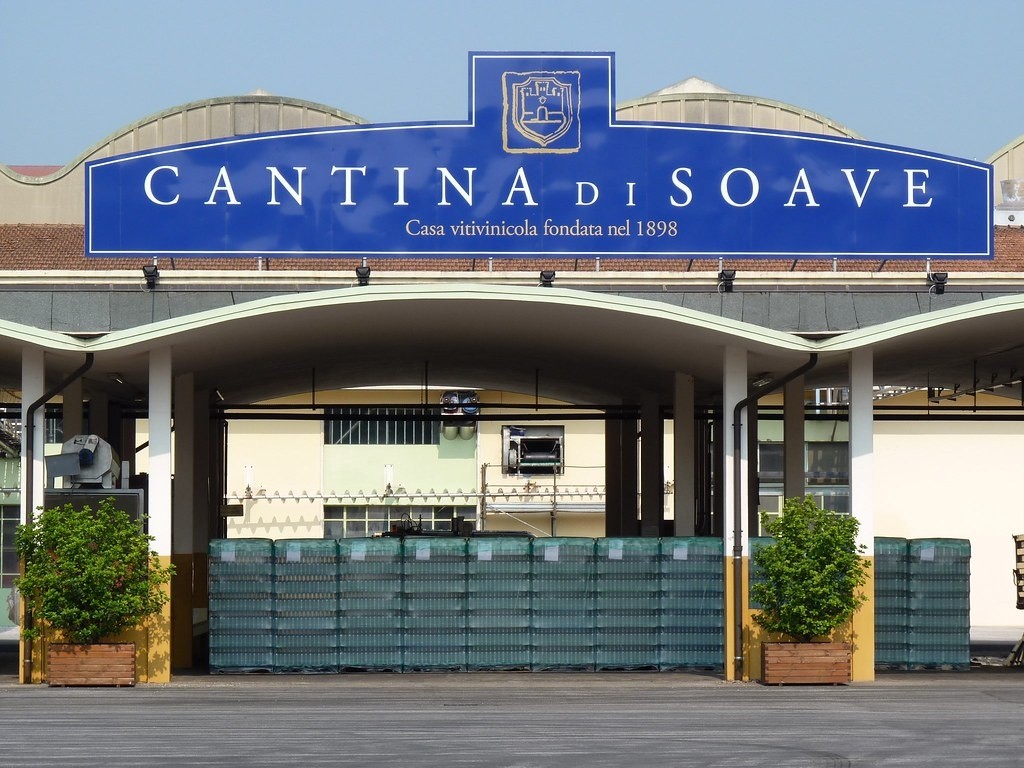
[14,496,176,684]
[746,493,872,682]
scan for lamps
[718,268,736,292]
[927,272,949,294]
[355,266,370,286]
[143,265,159,288]
[537,269,556,287]
